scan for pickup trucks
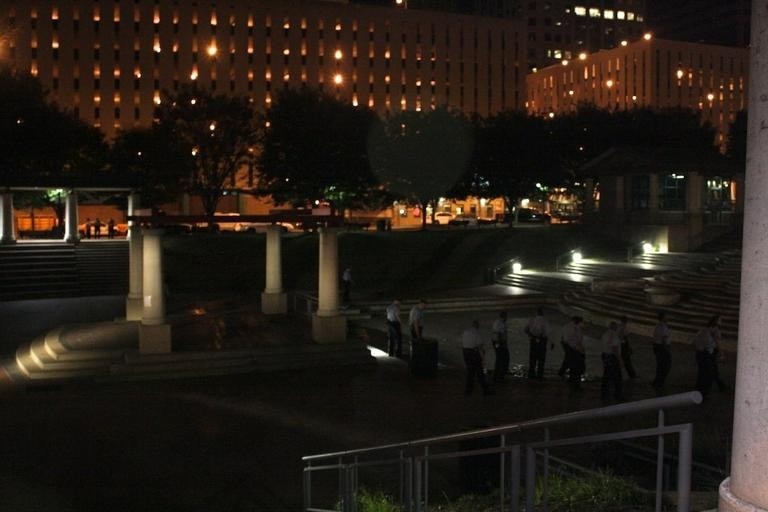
[496,208,551,224]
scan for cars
[78,220,119,237]
[115,222,128,235]
[244,218,293,233]
[298,215,370,231]
[421,211,457,225]
[457,213,478,224]
[552,208,567,219]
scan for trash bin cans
[411,336,438,377]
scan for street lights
[539,186,548,214]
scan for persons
[695,314,721,401]
[386,296,428,359]
[524,307,550,381]
[463,320,489,400]
[654,310,674,397]
[557,312,637,400]
[491,311,512,377]
[86,216,115,240]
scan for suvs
[174,209,242,233]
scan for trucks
[134,206,167,217]
